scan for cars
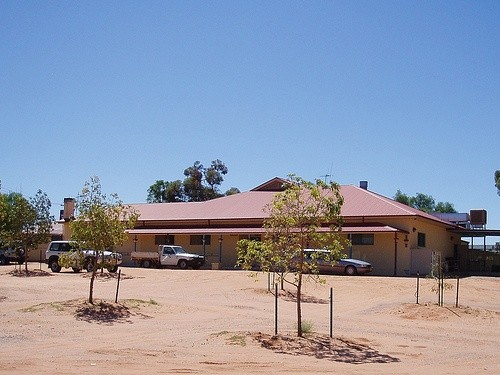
[289,249,373,276]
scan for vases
[211,262,224,270]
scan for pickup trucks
[131,244,208,269]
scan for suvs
[45,241,122,273]
[0,240,29,265]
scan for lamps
[404,235,409,248]
